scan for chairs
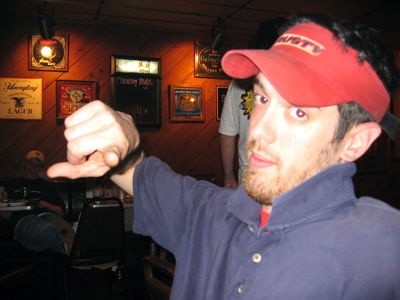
[66,196,125,300]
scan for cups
[20,186,30,202]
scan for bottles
[0,186,8,203]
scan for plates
[7,203,16,206]
[0,203,9,207]
[16,200,29,205]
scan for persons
[47,11,400,300]
[0,150,76,300]
[219,16,292,190]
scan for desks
[0,198,41,212]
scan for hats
[220,22,391,123]
[26,150,44,162]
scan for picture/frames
[28,33,69,72]
[194,37,227,78]
[169,83,204,122]
[55,79,97,120]
[216,85,228,120]
